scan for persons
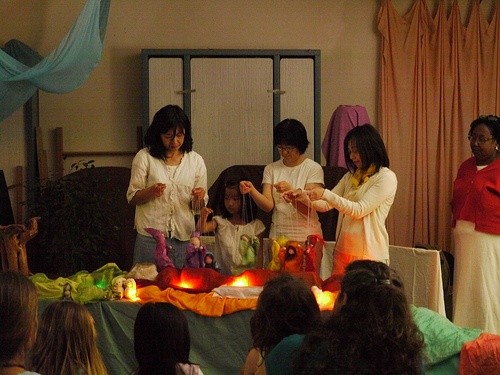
[239,119,325,262]
[255,260,427,375]
[197,176,266,274]
[0,271,43,375]
[239,234,261,267]
[129,302,204,375]
[449,115,500,334]
[183,232,220,271]
[30,299,107,375]
[285,124,397,276]
[144,228,175,268]
[241,272,321,375]
[269,235,325,271]
[127,104,210,269]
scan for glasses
[277,144,295,152]
[468,132,495,144]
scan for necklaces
[0,364,26,368]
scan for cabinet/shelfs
[143,48,322,195]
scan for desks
[33,295,333,375]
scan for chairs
[201,236,446,319]
